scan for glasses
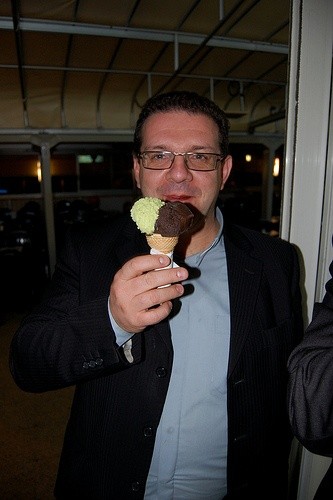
[135,149,226,171]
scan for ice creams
[130,197,195,305]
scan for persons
[287,231,333,500]
[8,92,304,500]
